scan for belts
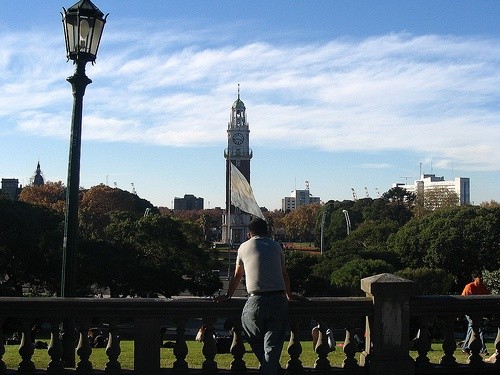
[247,291,288,297]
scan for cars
[163,340,179,349]
[7,338,21,345]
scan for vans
[233,243,242,249]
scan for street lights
[52,0,110,298]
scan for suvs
[88,328,120,348]
[213,331,233,353]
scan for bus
[211,242,232,250]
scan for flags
[231,163,268,221]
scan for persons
[461,270,490,353]
[226,218,292,375]
